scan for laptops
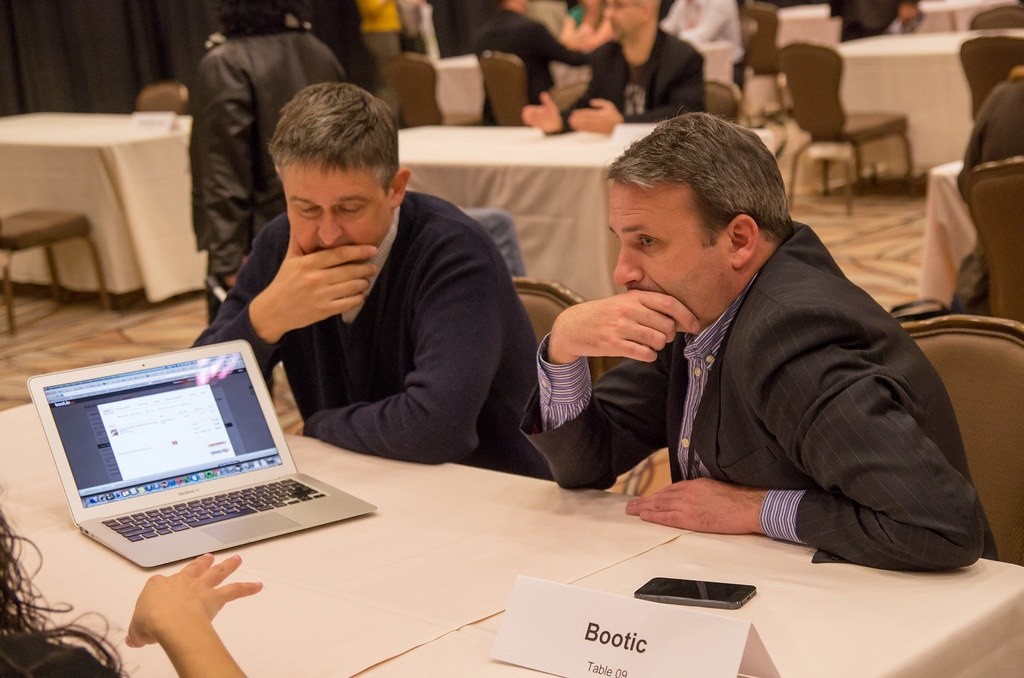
[26,339,377,570]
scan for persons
[187,82,552,480]
[472,0,746,135]
[0,511,265,678]
[190,0,401,323]
[953,66,1024,317]
[518,111,999,574]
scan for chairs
[374,0,1024,565]
[135,78,189,113]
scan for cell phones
[634,577,756,609]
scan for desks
[889,2,1007,35]
[396,122,776,310]
[831,26,1024,172]
[1,111,208,301]
[3,402,1024,678]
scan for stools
[0,209,110,335]
